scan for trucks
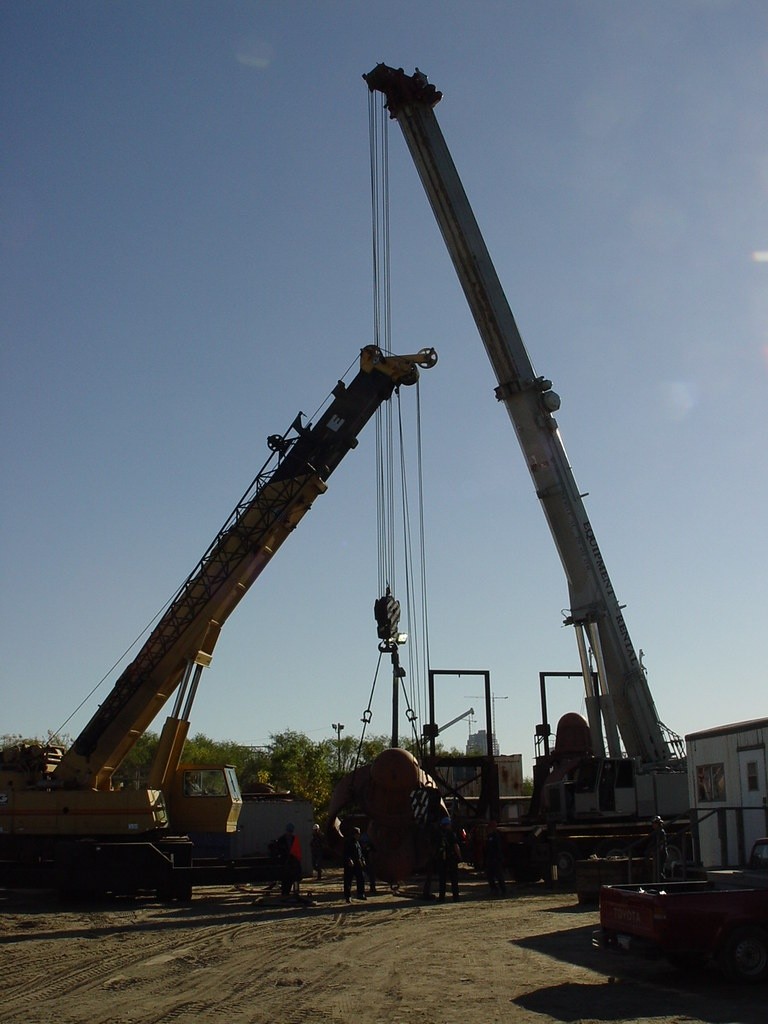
[598,837,768,986]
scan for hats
[490,821,498,830]
[313,824,319,829]
[441,817,451,824]
[650,815,664,823]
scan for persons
[310,824,326,881]
[434,817,461,902]
[338,827,378,904]
[276,823,305,896]
[647,815,666,882]
[483,820,506,897]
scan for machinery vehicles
[1,344,438,910]
[353,60,768,1023]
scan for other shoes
[491,888,498,896]
[346,897,351,903]
[439,899,444,903]
[501,888,507,897]
[357,895,366,900]
[454,899,458,901]
